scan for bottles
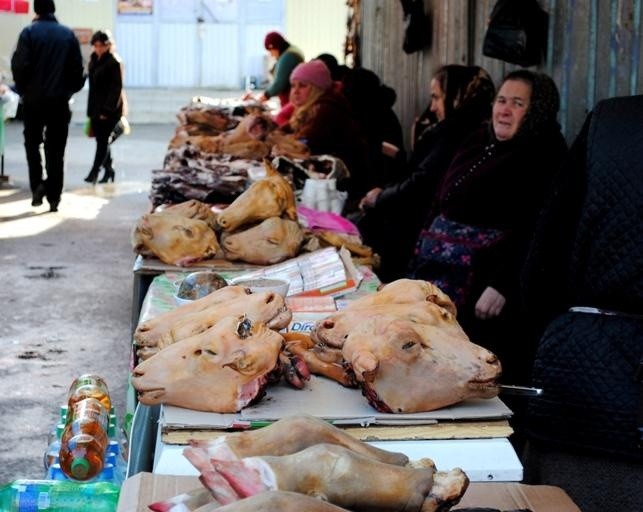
[0,479,122,511]
[47,372,132,480]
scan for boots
[85,159,114,183]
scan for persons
[10,1,85,217]
[240,29,403,218]
[399,69,572,459]
[346,60,495,287]
[81,31,128,186]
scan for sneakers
[32,179,57,211]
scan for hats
[290,60,332,91]
[265,33,284,50]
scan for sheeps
[131,284,292,360]
[130,175,304,267]
[130,315,286,413]
[310,278,457,362]
[341,312,502,414]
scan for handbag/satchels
[410,216,503,309]
[531,307,643,418]
